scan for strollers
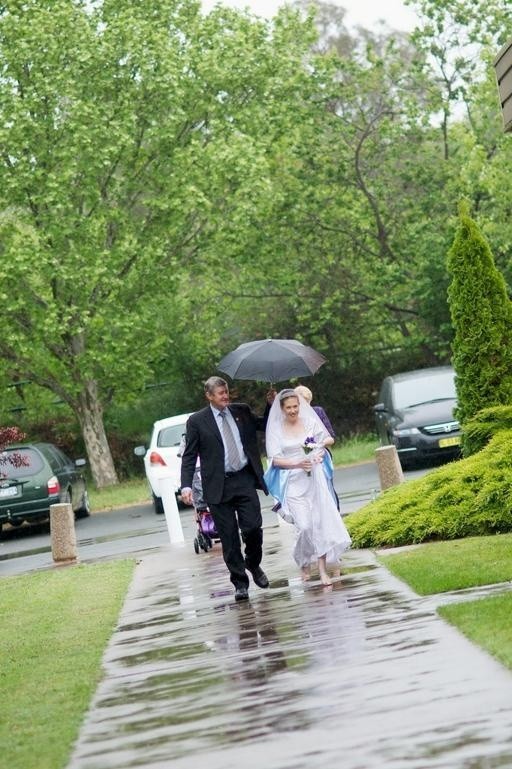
[190,465,222,554]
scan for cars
[134,411,201,514]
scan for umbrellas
[215,339,329,391]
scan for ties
[219,411,241,471]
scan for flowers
[302,434,316,477]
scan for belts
[224,472,234,478]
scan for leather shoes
[319,574,332,585]
[244,561,269,588]
[235,586,248,600]
[302,567,310,580]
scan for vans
[370,366,462,470]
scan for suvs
[1,440,91,531]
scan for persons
[295,384,340,514]
[264,387,352,586]
[181,377,277,599]
